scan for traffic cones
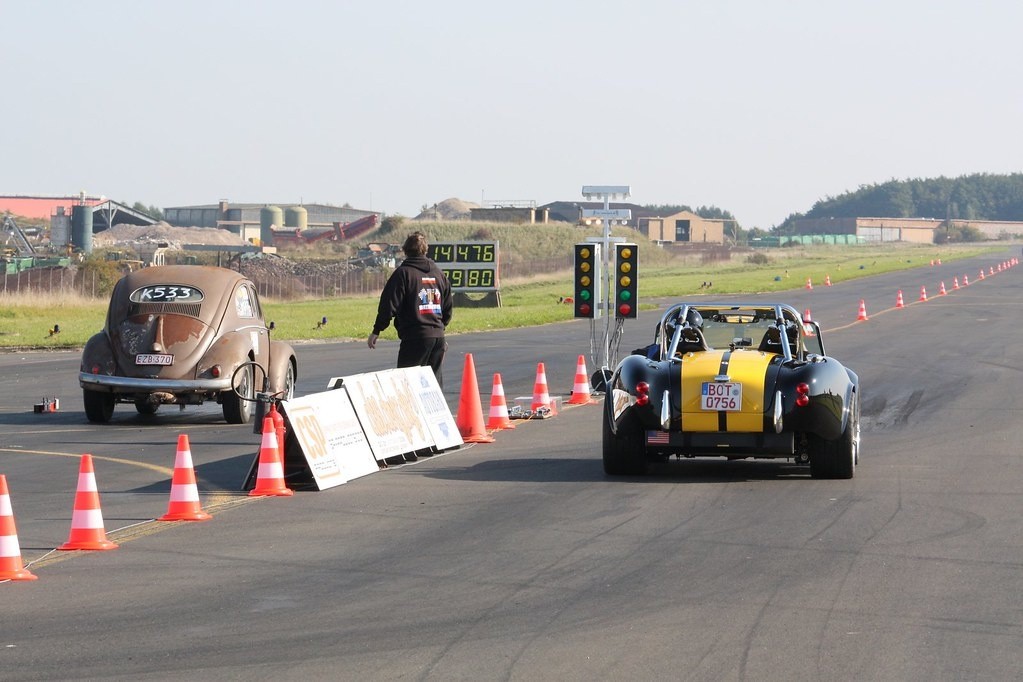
[952,276,960,289]
[936,259,941,265]
[919,285,928,301]
[930,258,934,267]
[0,474,38,580]
[564,354,598,405]
[803,309,815,336]
[531,362,556,416]
[938,280,947,295]
[825,275,832,286]
[54,452,121,551]
[979,268,985,279]
[157,435,213,521]
[247,417,294,496]
[895,290,905,307]
[455,354,496,443]
[857,300,869,320]
[996,258,1019,272]
[485,374,515,429]
[806,276,813,290]
[962,274,968,285]
[990,266,994,275]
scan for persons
[646,307,706,362]
[367,232,455,390]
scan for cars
[79,263,298,425]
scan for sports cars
[603,303,861,480]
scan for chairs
[669,328,708,355]
[759,329,799,356]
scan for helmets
[669,309,703,332]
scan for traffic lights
[614,245,637,319]
[572,242,600,319]
